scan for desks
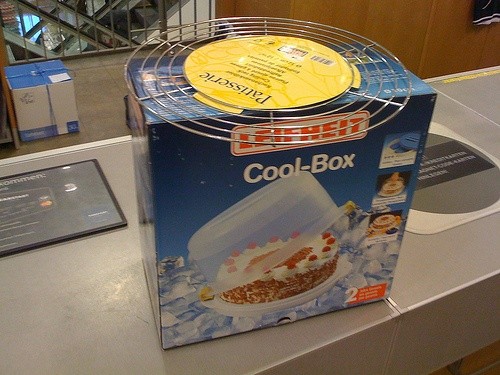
[2,66,500,375]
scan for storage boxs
[128,44,438,352]
[4,59,79,141]
